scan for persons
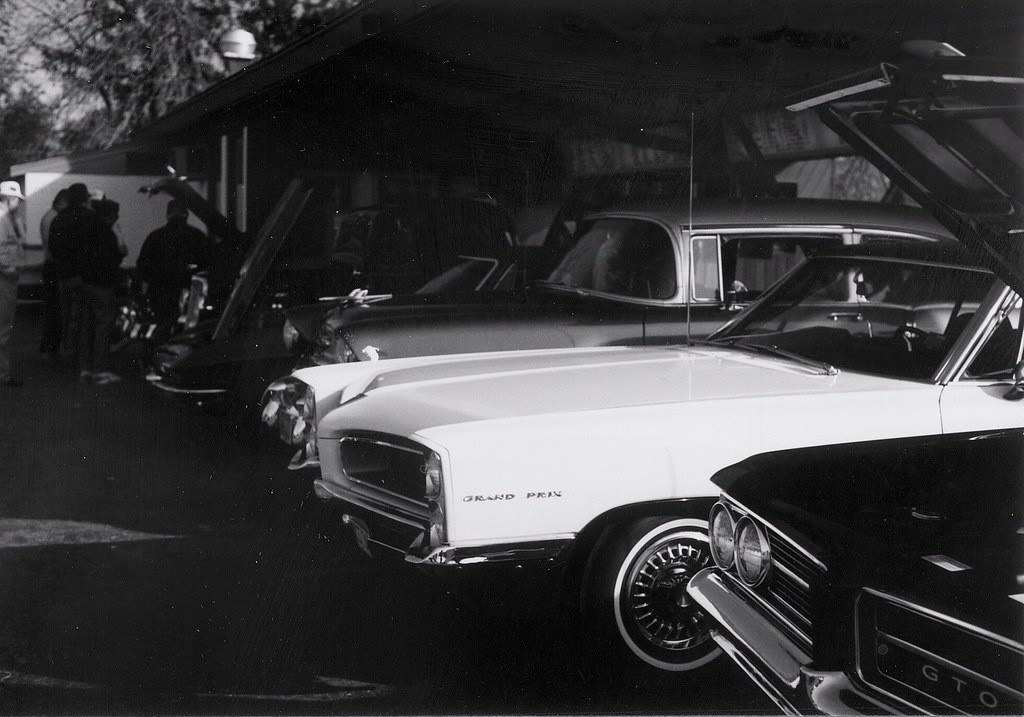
[77,197,126,384]
[38,188,71,365]
[1,177,33,388]
[47,182,95,356]
[136,195,208,380]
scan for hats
[1,180,27,203]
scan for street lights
[217,25,260,237]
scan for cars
[100,31,1023,714]
